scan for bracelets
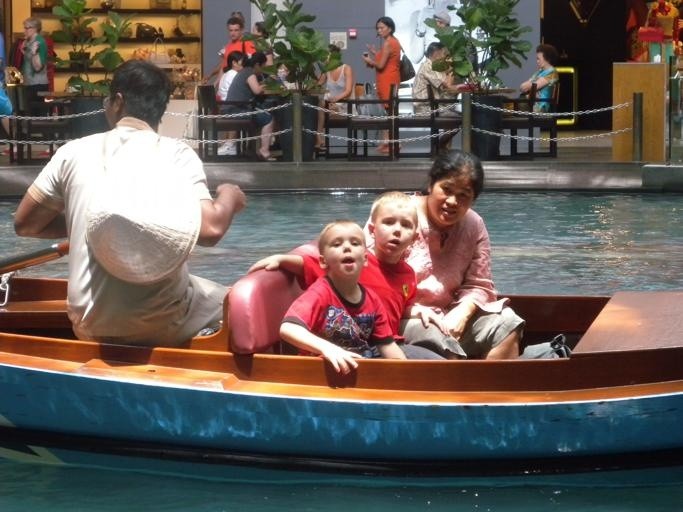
[366,60,373,67]
[31,54,36,57]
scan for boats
[0,274,683,459]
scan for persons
[19,18,48,117]
[502,44,559,118]
[200,9,359,162]
[432,12,478,110]
[648,1,679,64]
[12,59,247,348]
[361,149,526,360]
[362,17,402,154]
[245,191,449,360]
[0,32,15,139]
[278,219,406,375]
[411,42,461,150]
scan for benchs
[226,238,324,355]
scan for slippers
[315,143,328,150]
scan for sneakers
[216,142,237,155]
[375,142,402,154]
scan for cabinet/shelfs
[31,0,203,115]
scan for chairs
[9,83,560,164]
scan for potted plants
[417,0,534,161]
[46,0,138,140]
[240,1,343,163]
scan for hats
[433,11,451,25]
[83,170,204,287]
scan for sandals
[256,151,279,162]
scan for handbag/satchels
[113,22,159,41]
[131,37,170,65]
[400,45,415,82]
